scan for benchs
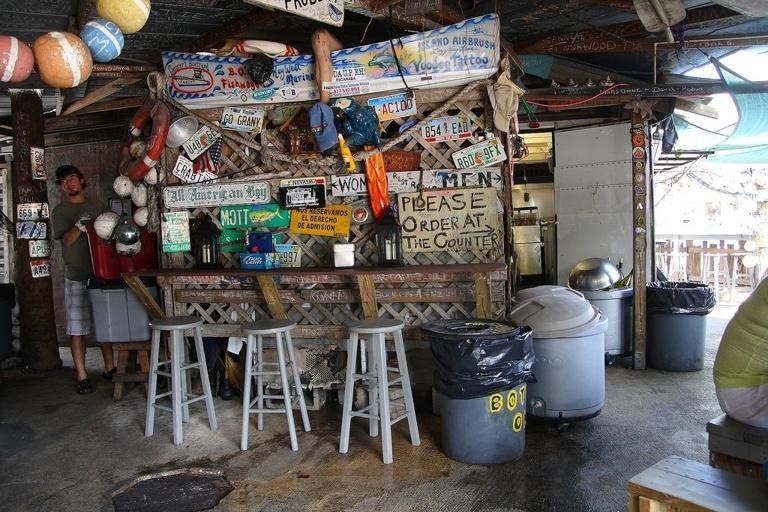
[705,412,768,475]
[627,453,768,511]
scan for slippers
[104,367,116,379]
[77,379,92,393]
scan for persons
[713,276,767,431]
[50,164,118,393]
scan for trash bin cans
[645,280,716,373]
[421,315,535,466]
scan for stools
[110,343,153,402]
[239,318,312,454]
[143,314,218,447]
[338,319,422,466]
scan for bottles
[337,132,357,172]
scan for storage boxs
[86,282,159,342]
[79,217,156,279]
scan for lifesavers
[118,98,169,179]
[233,39,298,57]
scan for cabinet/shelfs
[513,224,557,286]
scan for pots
[568,257,622,291]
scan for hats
[309,103,338,152]
